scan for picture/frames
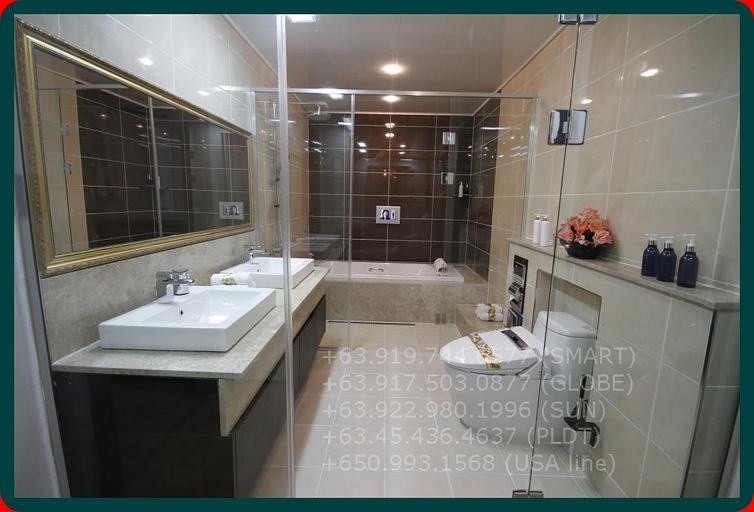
[376,206,401,225]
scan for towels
[474,303,503,321]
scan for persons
[229,206,239,215]
[380,210,390,220]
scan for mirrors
[14,16,257,277]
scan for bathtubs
[316,260,463,278]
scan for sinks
[293,243,330,252]
[223,257,315,287]
[99,284,277,352]
[310,233,341,242]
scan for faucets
[243,242,268,263]
[155,270,194,296]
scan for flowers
[553,207,615,248]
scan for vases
[564,240,600,258]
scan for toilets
[438,310,596,441]
